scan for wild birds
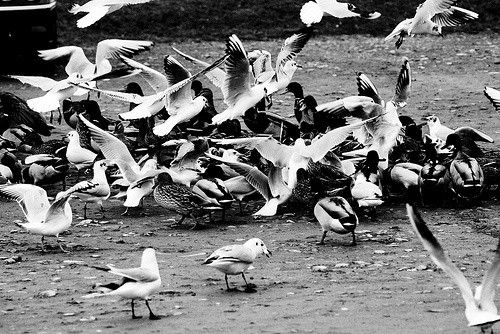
[403,202,500,334]
[201,237,272,293]
[86,248,163,321]
[0,0,500,252]
